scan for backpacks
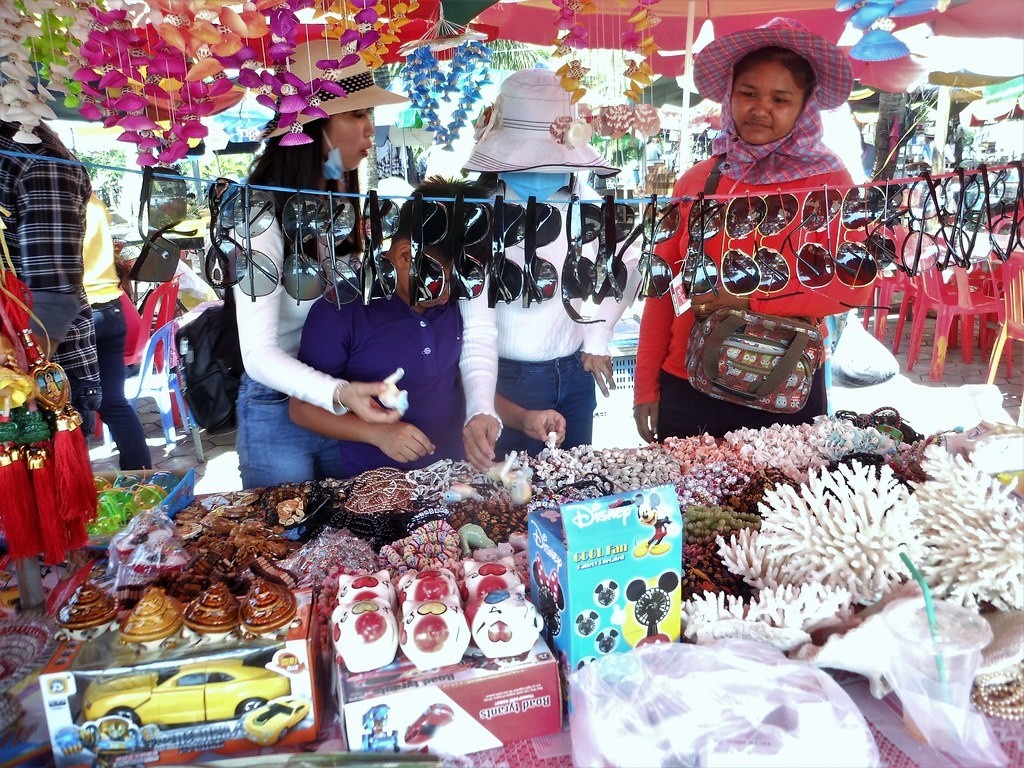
[173,304,248,435]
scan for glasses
[126,158,1024,303]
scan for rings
[700,304,706,313]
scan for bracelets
[336,383,351,411]
[378,520,460,579]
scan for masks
[321,130,347,183]
[497,172,572,200]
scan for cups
[884,596,991,741]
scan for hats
[694,17,854,111]
[458,68,622,181]
[249,39,410,146]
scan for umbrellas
[464,0,1024,226]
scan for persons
[0,119,102,446]
[633,17,874,444]
[225,40,410,490]
[458,68,644,472]
[288,175,566,478]
[81,164,151,471]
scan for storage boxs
[605,356,637,395]
[323,630,565,757]
[528,482,683,724]
[39,587,327,768]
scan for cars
[82,639,294,731]
[403,702,456,746]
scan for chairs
[862,204,1024,384]
[95,280,182,440]
[102,315,189,450]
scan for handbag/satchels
[685,308,826,416]
